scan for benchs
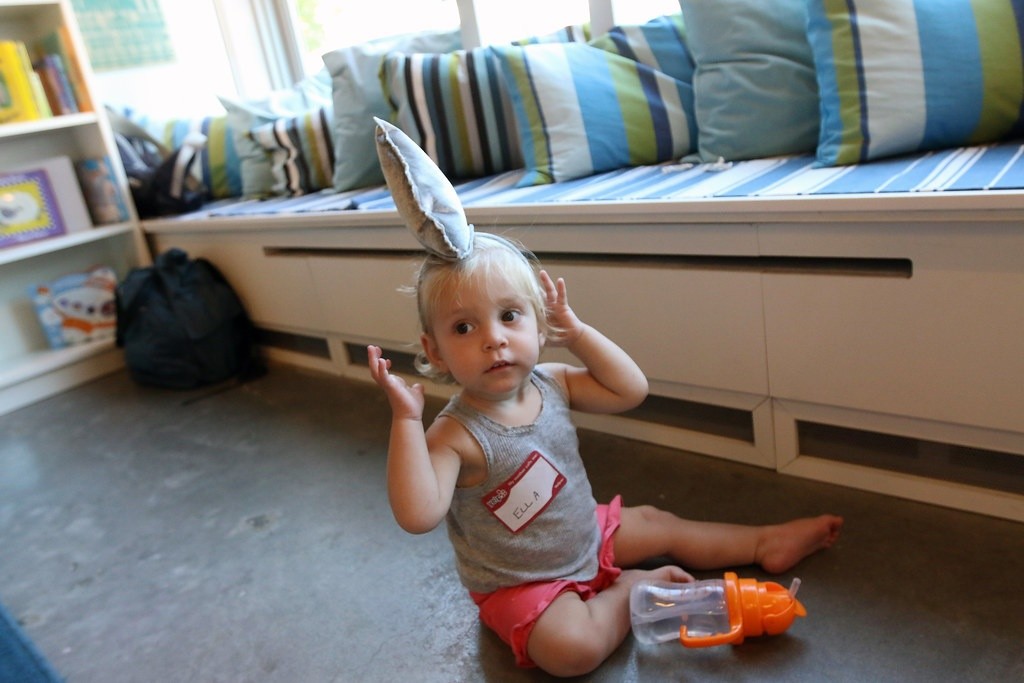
[143,134,1024,526]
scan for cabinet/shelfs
[0,1,158,422]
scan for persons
[366,232,842,677]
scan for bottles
[629,571,808,648]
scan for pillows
[105,67,331,212]
[804,0,1024,168]
[241,99,335,203]
[377,20,599,180]
[681,0,821,163]
[490,15,697,186]
[320,24,466,194]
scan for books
[0,27,93,124]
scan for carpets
[0,604,63,683]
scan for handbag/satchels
[114,249,265,391]
